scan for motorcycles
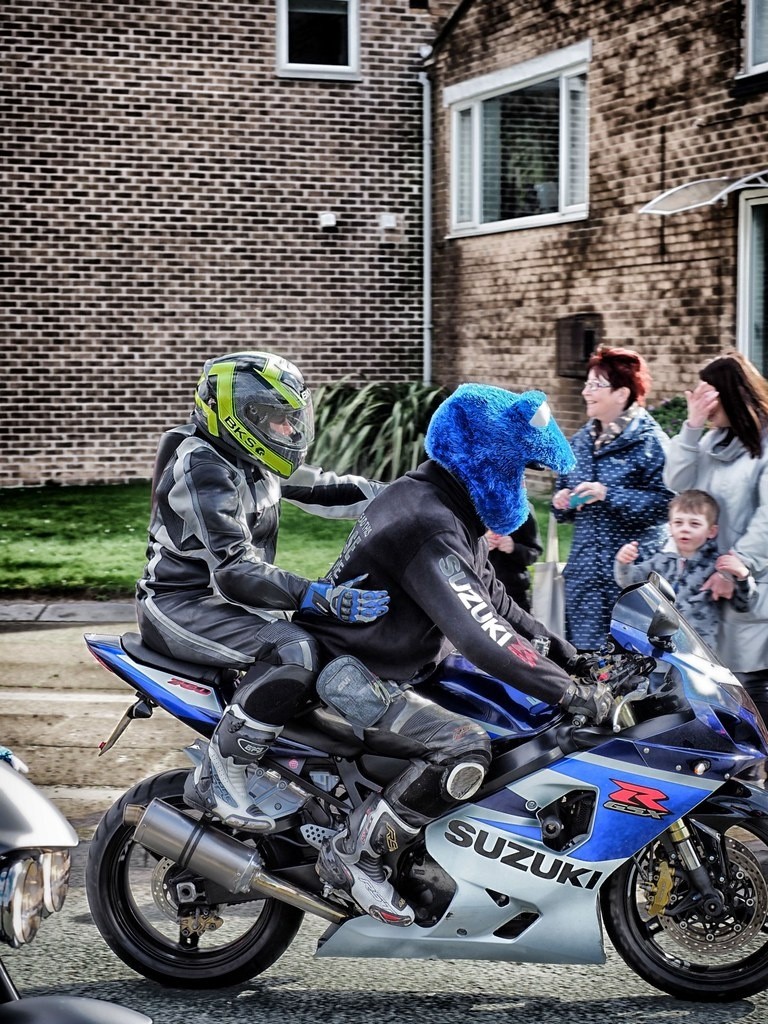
[83,568,768,1002]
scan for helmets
[424,382,576,537]
[192,351,315,480]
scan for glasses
[267,410,300,426]
[585,380,613,390]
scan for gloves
[558,680,615,725]
[565,652,599,678]
[299,573,390,623]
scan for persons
[546,343,670,653]
[136,352,394,840]
[613,488,760,658]
[661,353,768,704]
[293,383,637,927]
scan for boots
[183,703,284,833]
[315,793,422,927]
